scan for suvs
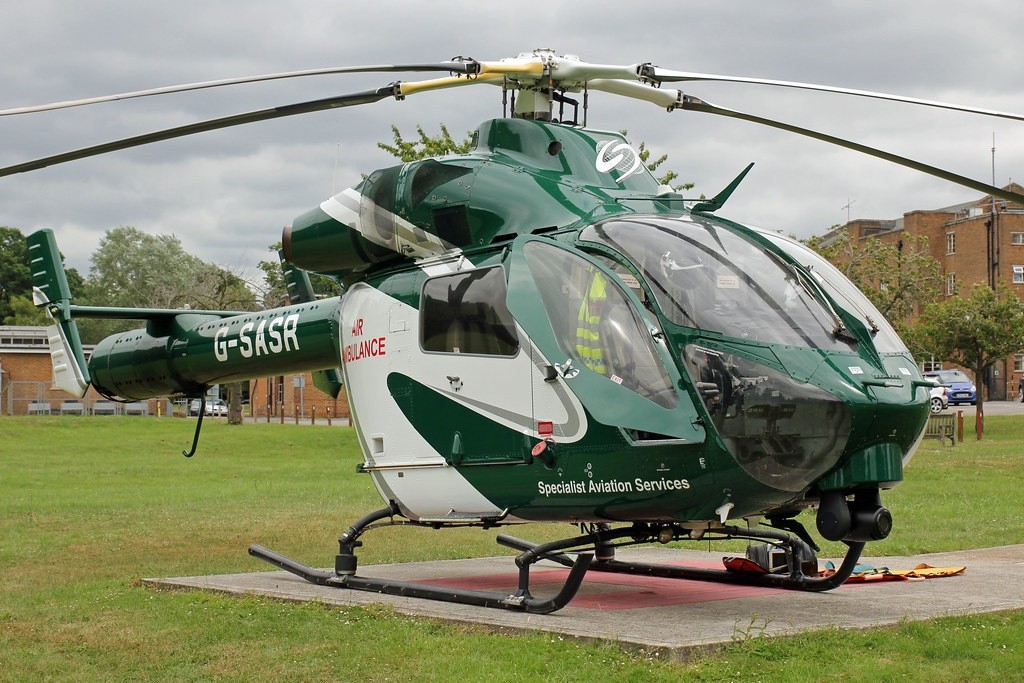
[930,370,977,407]
[922,372,948,414]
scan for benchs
[60,403,84,415]
[91,403,116,415]
[27,402,51,415]
[123,403,149,416]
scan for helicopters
[0,48,1024,615]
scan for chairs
[445,288,501,355]
[698,265,788,376]
[599,262,698,395]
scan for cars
[1017,374,1024,403]
[190,397,229,417]
